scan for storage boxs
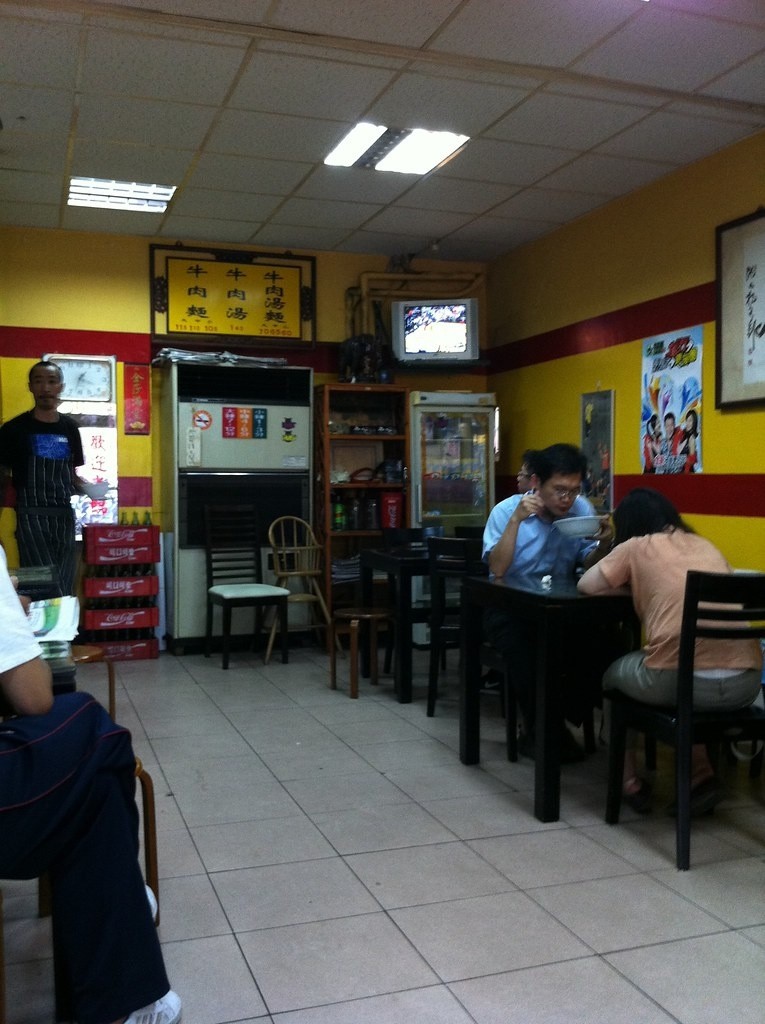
[81,523,161,661]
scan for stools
[323,599,394,699]
[66,646,115,727]
[37,749,164,924]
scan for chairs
[197,499,297,672]
[261,514,350,668]
[597,568,765,875]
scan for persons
[577,486,763,821]
[0,360,92,603]
[682,409,699,473]
[643,414,663,473]
[0,544,182,1024]
[481,442,615,768]
[657,412,687,473]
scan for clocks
[42,353,117,403]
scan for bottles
[87,511,153,640]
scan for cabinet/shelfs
[314,379,412,655]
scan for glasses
[548,480,582,498]
[518,471,530,478]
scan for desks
[425,534,510,722]
[359,538,466,704]
[447,567,643,823]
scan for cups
[332,492,403,530]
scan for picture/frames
[577,386,616,517]
[712,205,765,413]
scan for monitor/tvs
[391,298,479,361]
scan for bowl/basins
[553,516,604,540]
[82,482,108,499]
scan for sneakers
[480,669,500,694]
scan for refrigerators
[409,390,495,604]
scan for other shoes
[624,775,650,804]
[145,885,157,922]
[517,724,587,764]
[668,776,725,815]
[121,989,182,1024]
